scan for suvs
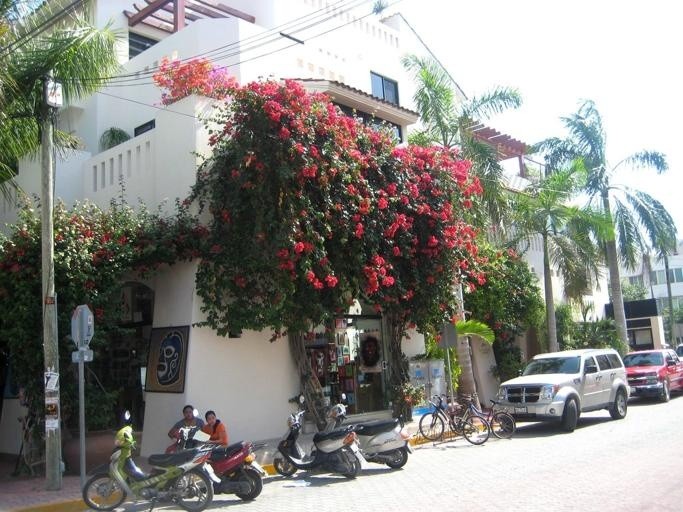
[495,347,632,432]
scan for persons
[197,411,227,447]
[166,405,205,454]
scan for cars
[622,348,682,402]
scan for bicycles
[420,394,491,445]
[448,391,516,440]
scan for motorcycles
[272,395,367,480]
[177,409,266,502]
[83,410,221,511]
[323,393,414,470]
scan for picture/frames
[143,326,189,395]
